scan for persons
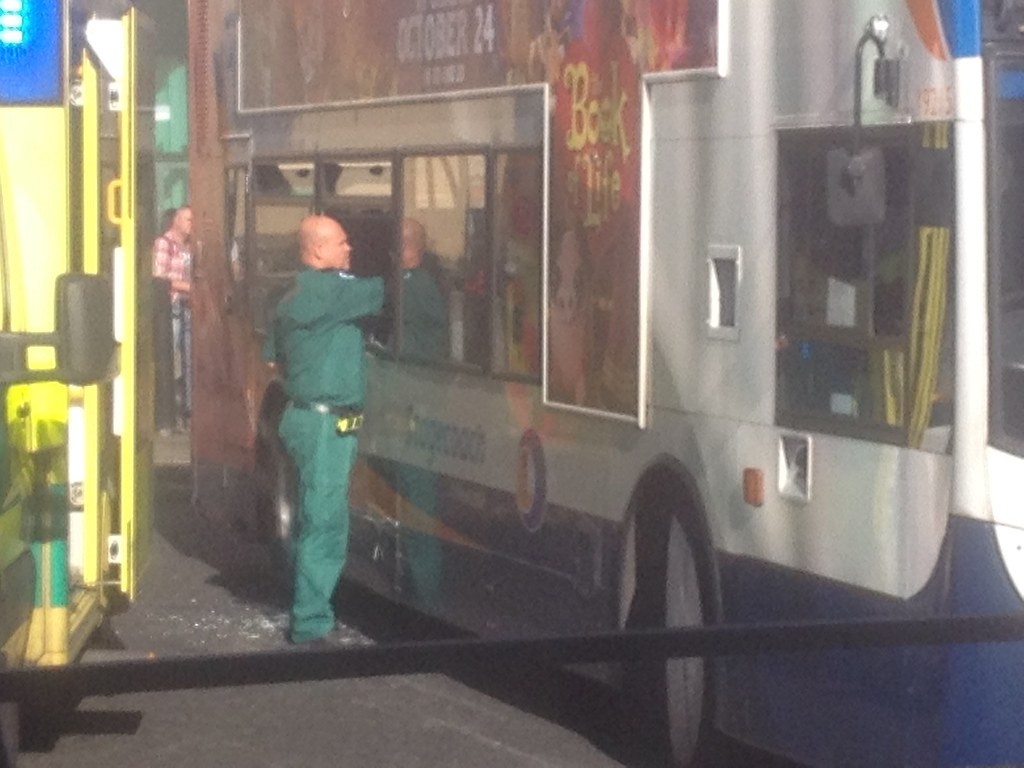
[397,225,439,362]
[261,217,382,646]
[151,206,192,435]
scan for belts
[292,400,354,417]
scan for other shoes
[300,628,360,647]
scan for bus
[0,1,164,760]
[186,0,1024,768]
